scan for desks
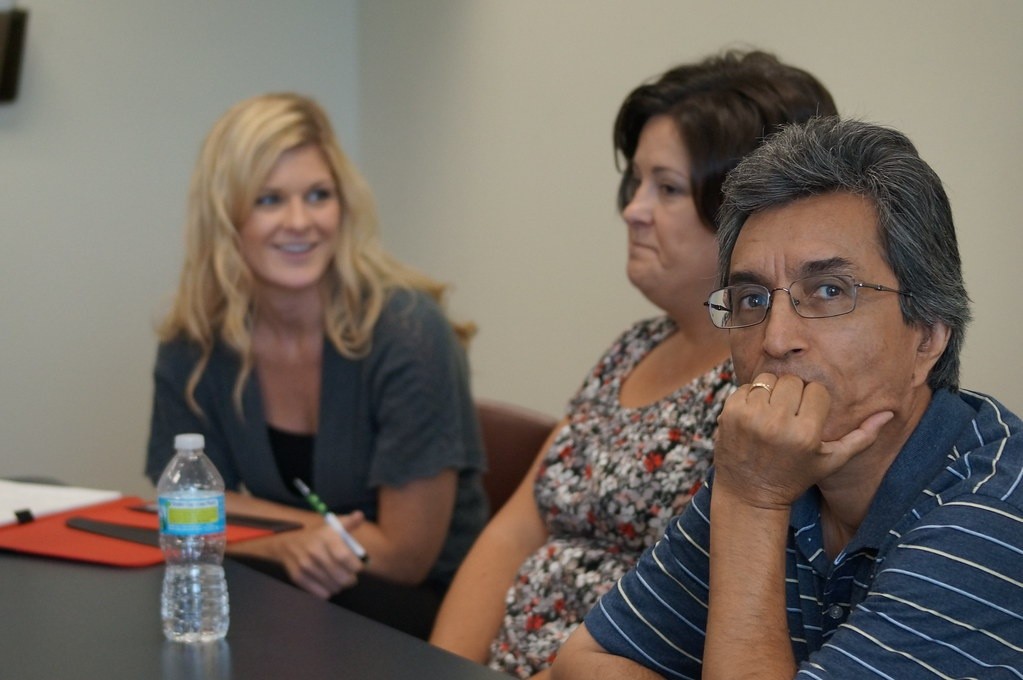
[0,478,522,680]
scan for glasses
[703,273,912,329]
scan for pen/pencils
[293,477,369,564]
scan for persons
[429,49,840,680]
[552,118,1023,680]
[143,94,490,642]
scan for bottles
[157,433,229,644]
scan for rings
[749,383,771,392]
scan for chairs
[450,399,559,528]
